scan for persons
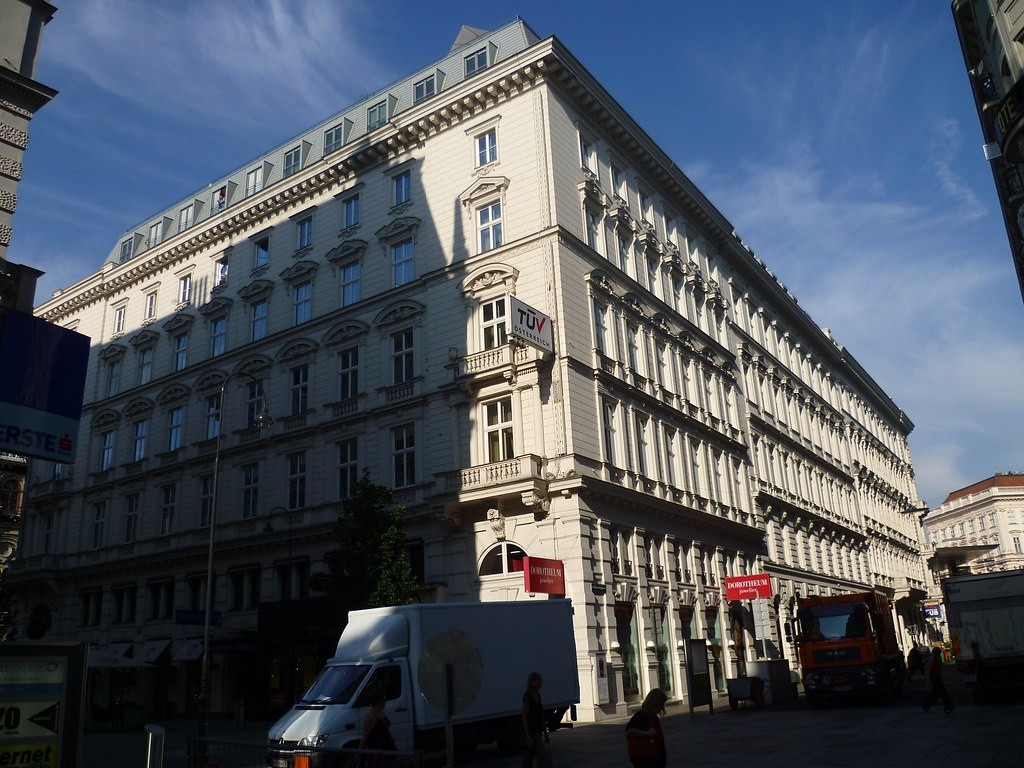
[922,647,955,714]
[353,695,400,768]
[908,642,925,677]
[728,611,747,678]
[522,673,550,768]
[624,689,667,768]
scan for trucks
[784,590,907,706]
[265,598,580,767]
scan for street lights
[264,506,293,601]
[199,373,279,738]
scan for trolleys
[726,676,770,708]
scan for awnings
[172,639,204,660]
[137,639,172,662]
[106,643,132,656]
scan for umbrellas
[88,653,158,667]
